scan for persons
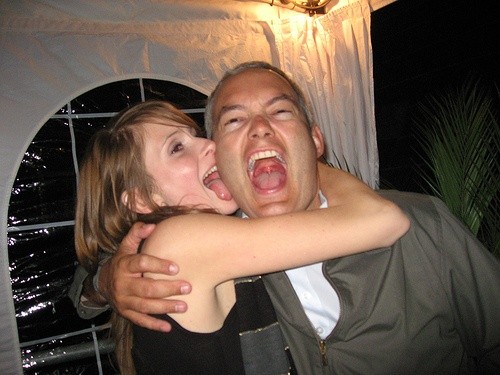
[67,60,500,375]
[73,100,411,375]
